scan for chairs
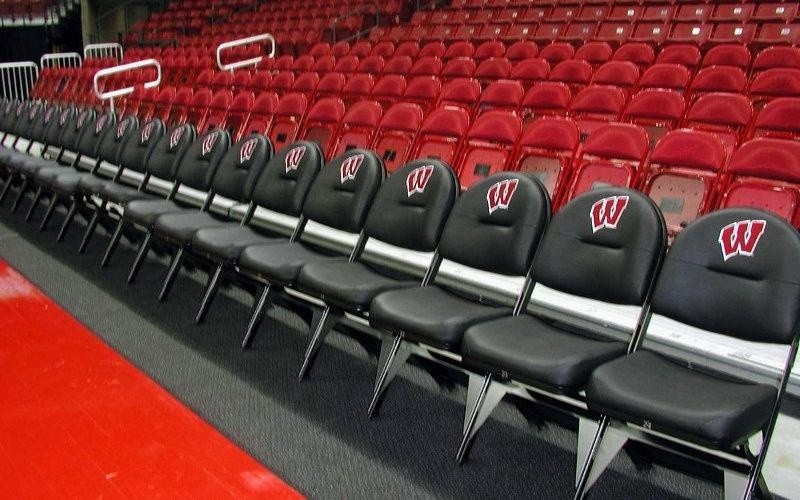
[444,42,474,57]
[223,90,255,148]
[241,90,278,149]
[453,186,668,500]
[446,24,481,45]
[505,41,538,58]
[501,23,537,45]
[747,67,800,96]
[639,129,728,253]
[544,6,580,22]
[152,134,274,324]
[102,124,197,284]
[558,21,599,48]
[549,59,592,85]
[125,128,233,303]
[53,115,139,253]
[705,23,757,51]
[682,91,753,178]
[471,80,523,124]
[446,0,800,8]
[490,8,526,24]
[574,206,799,499]
[589,60,639,90]
[752,23,799,55]
[31,0,406,103]
[422,10,454,26]
[239,149,386,386]
[410,56,442,75]
[372,104,423,178]
[36,112,117,242]
[445,10,476,25]
[202,89,233,137]
[298,158,461,420]
[465,9,500,24]
[437,78,481,120]
[749,3,798,23]
[539,43,574,60]
[708,4,755,23]
[701,44,752,68]
[626,22,672,53]
[400,25,427,43]
[442,57,475,75]
[0,102,46,214]
[2,0,81,25]
[639,64,690,91]
[267,91,307,155]
[456,110,522,197]
[409,104,470,181]
[404,10,432,27]
[0,98,20,121]
[572,5,611,23]
[19,108,97,232]
[746,98,800,142]
[527,23,567,46]
[715,137,800,231]
[664,22,714,53]
[420,42,446,57]
[620,87,685,168]
[604,5,644,24]
[690,65,748,91]
[423,25,457,44]
[366,172,552,468]
[613,43,654,65]
[474,41,505,58]
[0,100,31,145]
[472,25,508,45]
[559,121,649,221]
[192,141,325,352]
[574,42,612,62]
[517,7,552,25]
[655,43,702,68]
[299,96,345,165]
[401,75,441,118]
[753,46,800,68]
[569,83,625,158]
[638,5,678,23]
[522,80,571,132]
[473,57,509,78]
[511,58,549,81]
[589,22,635,53]
[511,115,581,214]
[672,4,715,24]
[6,104,62,222]
[330,101,382,172]
[79,118,167,268]
